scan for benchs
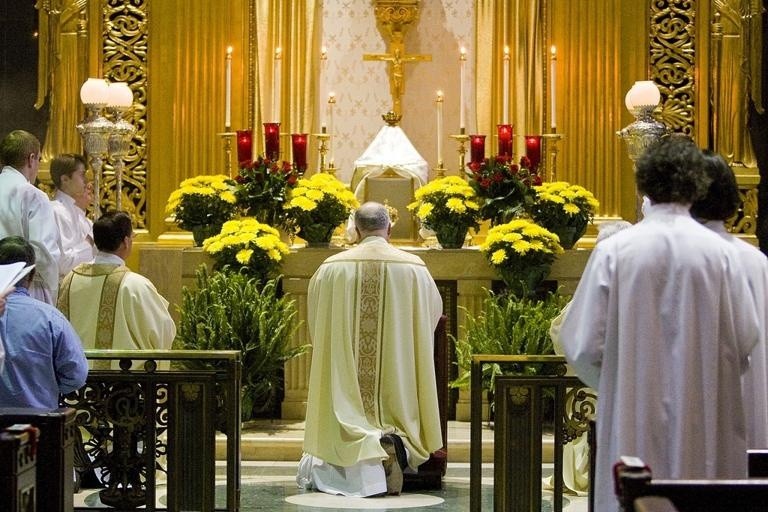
[0,408,77,512]
[609,455,768,511]
[0,423,40,512]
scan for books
[0,261,37,291]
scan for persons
[74,175,96,233]
[557,133,764,512]
[48,152,96,285]
[542,220,633,503]
[0,235,89,408]
[0,287,14,316]
[0,129,62,309]
[686,146,765,459]
[55,209,177,371]
[293,201,446,499]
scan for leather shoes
[379,436,403,495]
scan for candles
[469,135,486,162]
[237,130,252,168]
[292,134,306,173]
[225,45,232,128]
[524,135,540,168]
[320,46,328,127]
[436,90,444,168]
[551,45,556,133]
[460,47,467,134]
[503,45,510,125]
[272,48,282,125]
[327,89,335,162]
[263,123,279,160]
[496,124,512,156]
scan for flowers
[234,159,296,207]
[281,173,359,242]
[479,219,565,276]
[465,156,542,214]
[531,181,600,231]
[407,176,481,227]
[164,174,236,232]
[202,218,291,285]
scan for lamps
[618,79,665,170]
[76,77,115,220]
[108,81,136,212]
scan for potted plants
[169,260,313,435]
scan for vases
[300,219,334,248]
[434,225,469,248]
[502,273,537,297]
[193,225,216,246]
[549,226,577,248]
